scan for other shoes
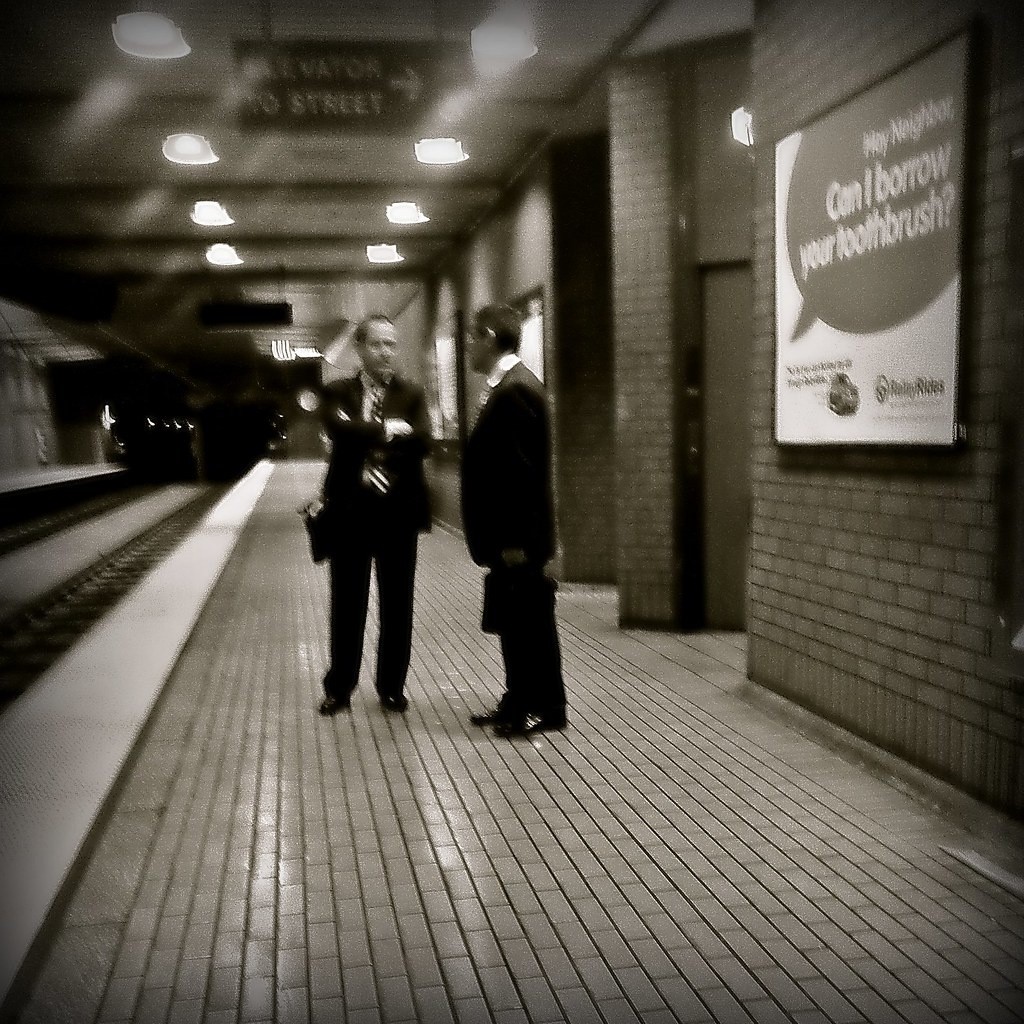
[321,693,351,713]
[380,693,407,711]
[494,704,566,736]
[469,700,503,724]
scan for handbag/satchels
[298,502,326,564]
[481,572,559,633]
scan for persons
[317,313,435,715]
[458,303,568,737]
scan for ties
[370,381,391,496]
